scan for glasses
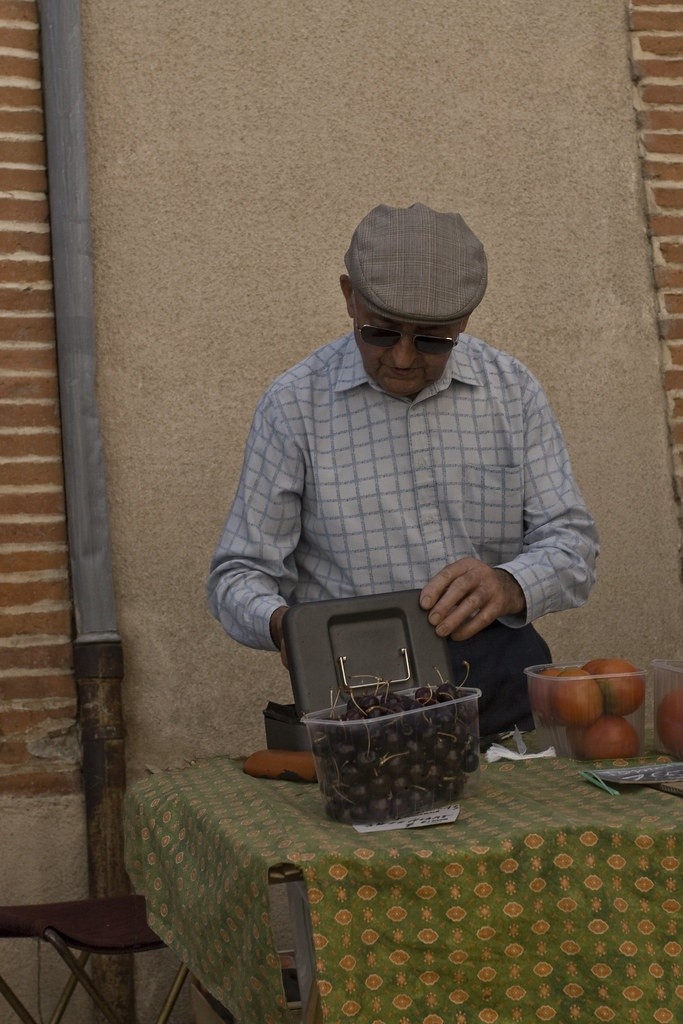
[352,289,463,355]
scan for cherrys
[312,659,480,824]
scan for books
[585,762,683,796]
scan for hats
[344,203,488,324]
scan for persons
[203,202,602,738]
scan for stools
[0,896,192,1024]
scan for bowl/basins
[523,662,648,759]
[300,686,481,824]
[650,658,683,761]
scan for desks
[121,729,683,1024]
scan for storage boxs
[523,661,649,760]
[651,658,683,764]
[299,684,483,825]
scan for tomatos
[530,657,645,760]
[655,684,683,759]
